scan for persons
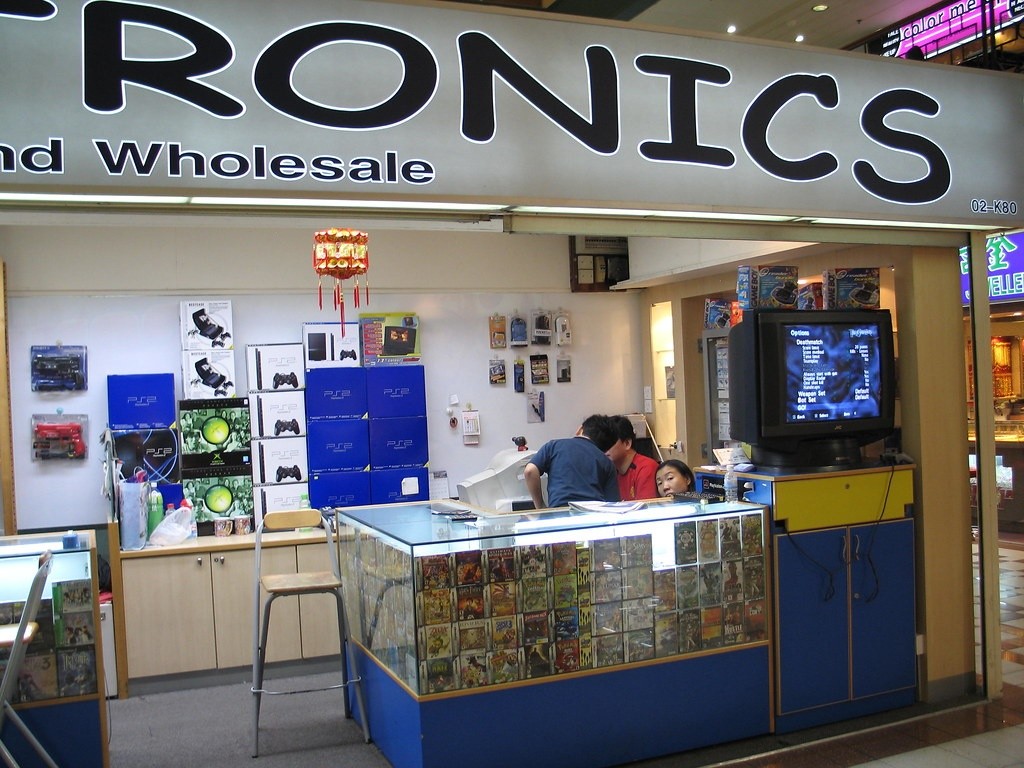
[524,414,660,510]
[655,459,695,498]
[183,477,253,519]
[181,409,250,454]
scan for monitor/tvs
[457,448,549,511]
[728,307,895,474]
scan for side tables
[771,518,918,734]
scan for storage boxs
[106,299,430,537]
[703,265,881,329]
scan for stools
[251,508,371,758]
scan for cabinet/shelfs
[335,497,774,768]
[121,541,340,680]
[0,529,111,768]
